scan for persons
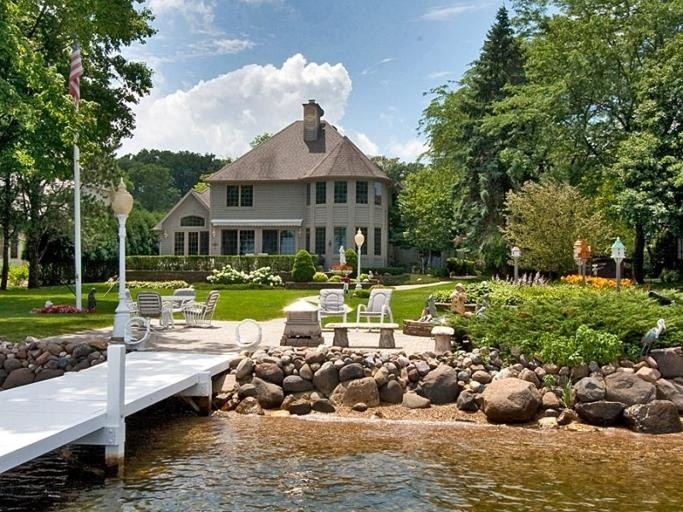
[478,280,494,298]
[340,274,349,294]
[88,287,97,310]
[449,283,467,315]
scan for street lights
[511,246,521,282]
[110,176,134,339]
[353,227,367,291]
[609,237,626,293]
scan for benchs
[323,320,398,348]
[429,326,455,352]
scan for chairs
[121,287,393,350]
